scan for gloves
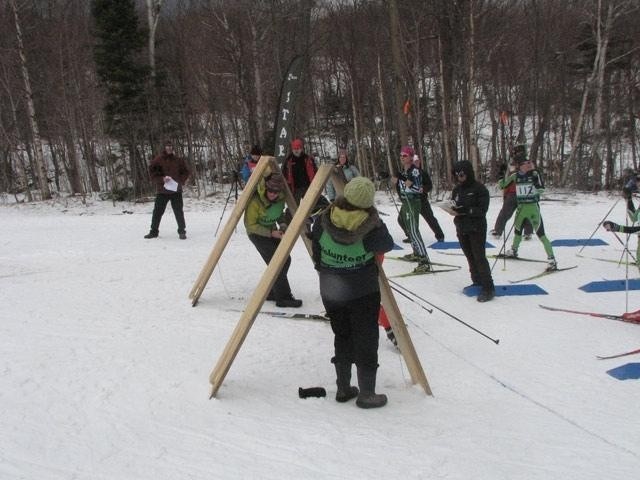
[531,170,538,181]
[395,172,407,182]
[623,186,632,202]
[452,206,470,215]
[499,164,507,174]
[603,221,619,232]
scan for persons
[391,145,430,274]
[326,151,360,204]
[397,154,444,243]
[241,146,262,184]
[144,140,187,239]
[490,143,534,240]
[498,159,558,271]
[243,172,303,308]
[281,138,318,226]
[448,160,495,303]
[311,175,395,408]
[603,186,640,321]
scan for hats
[291,139,303,149]
[250,144,262,155]
[343,176,376,209]
[400,147,414,156]
[266,175,284,191]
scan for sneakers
[179,233,186,240]
[506,249,518,257]
[144,230,158,239]
[265,287,302,307]
[404,254,419,262]
[402,238,411,243]
[488,229,501,237]
[545,257,557,272]
[414,257,430,273]
[524,233,532,240]
[463,284,496,302]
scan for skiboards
[540,304,640,359]
[384,255,461,280]
[485,254,577,285]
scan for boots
[335,361,359,402]
[355,365,387,409]
